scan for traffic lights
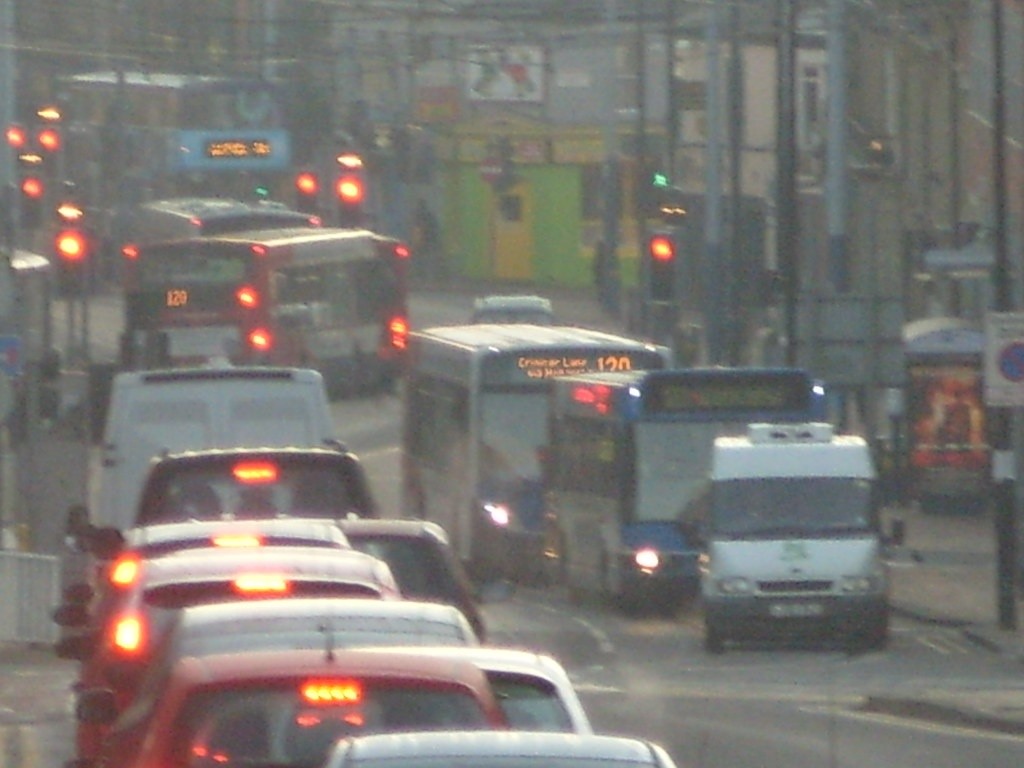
[5,122,34,155]
[29,127,62,161]
[53,185,93,270]
[335,178,365,231]
[295,172,315,217]
[649,231,675,301]
[19,168,51,203]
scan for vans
[700,421,910,648]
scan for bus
[539,363,828,621]
[111,226,417,399]
[135,200,317,256]
[56,71,291,207]
[397,322,672,596]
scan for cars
[42,358,684,768]
[463,294,563,328]
